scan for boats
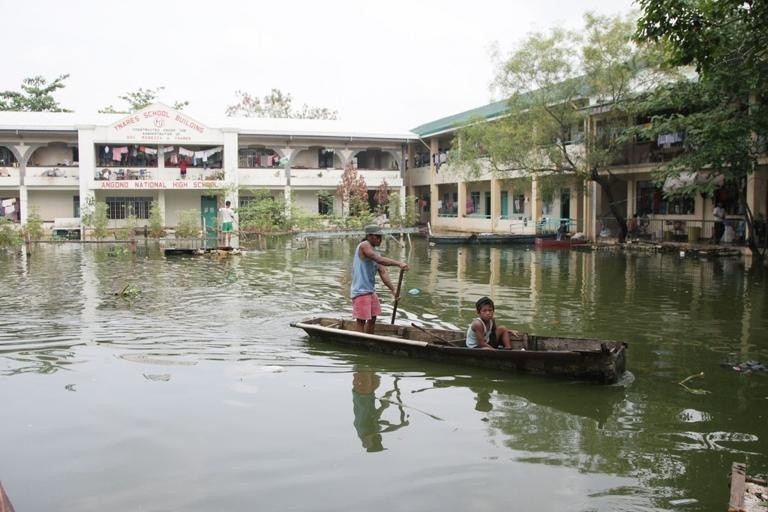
[427,233,596,252]
[164,245,245,256]
[289,315,628,385]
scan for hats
[364,225,386,236]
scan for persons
[466,296,520,350]
[351,225,409,335]
[557,221,567,241]
[670,221,684,235]
[712,201,726,243]
[180,158,187,179]
[217,201,234,246]
[629,213,638,238]
